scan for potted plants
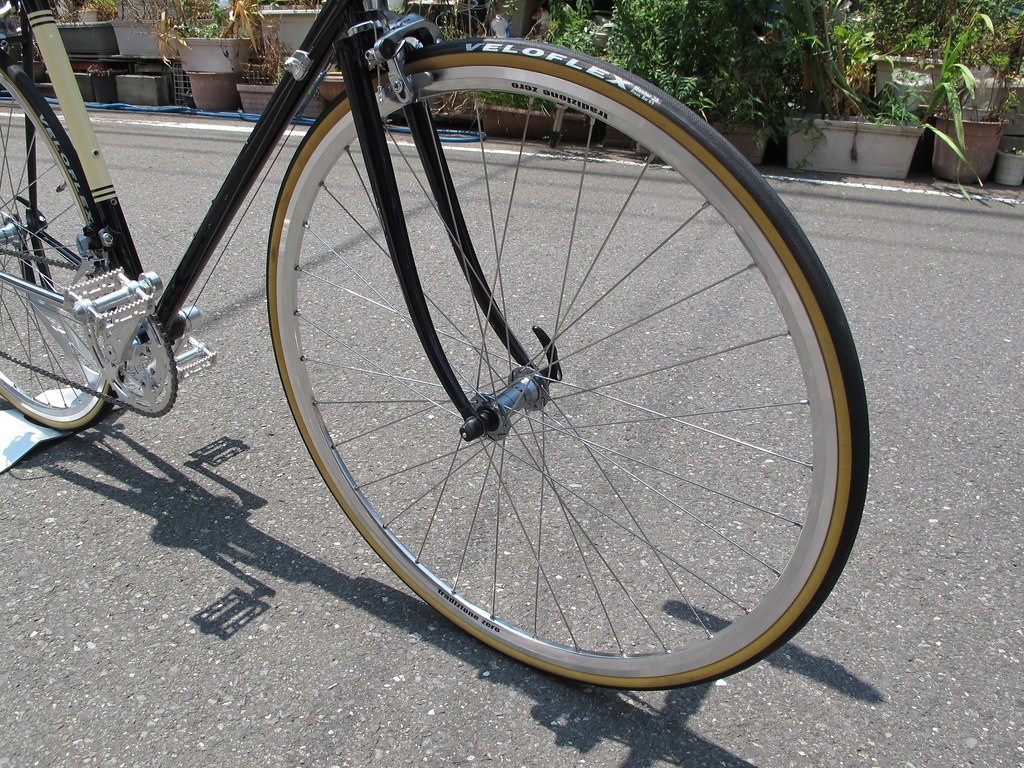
[56,1,1024,186]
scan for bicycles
[0,1,874,693]
